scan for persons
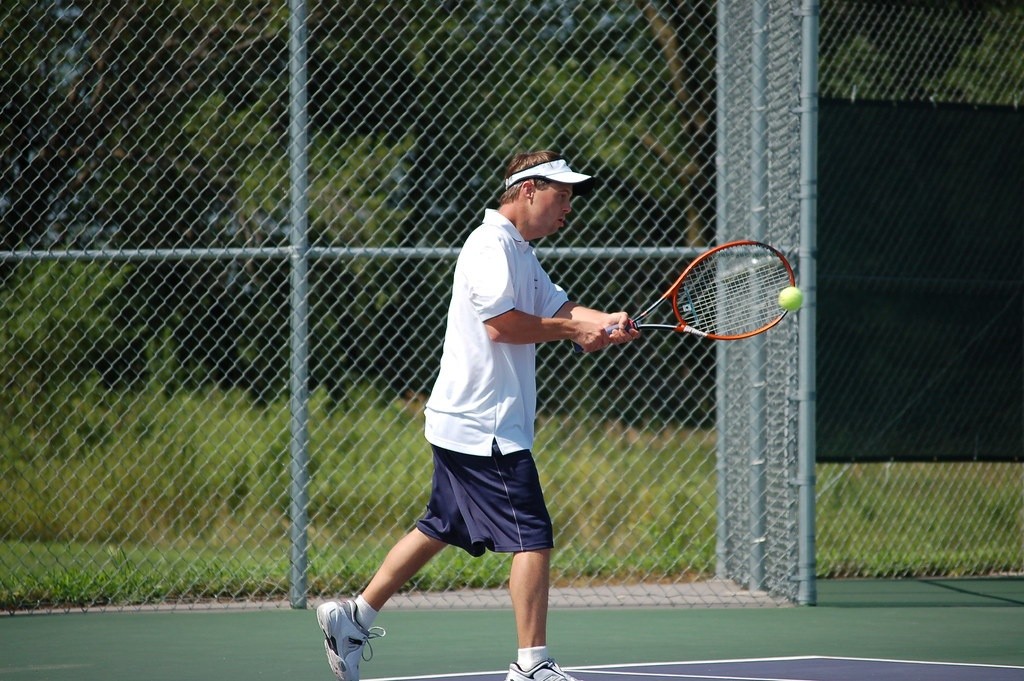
[317,151,640,681]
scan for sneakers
[506,657,584,681]
[317,600,386,681]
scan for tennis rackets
[571,239,795,354]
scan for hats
[505,159,592,190]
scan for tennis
[778,286,805,312]
